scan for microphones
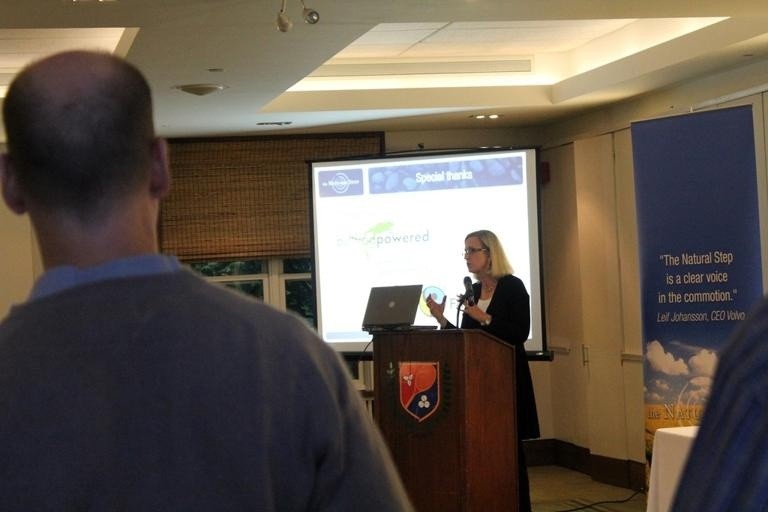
[464,277,474,306]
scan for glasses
[465,247,488,253]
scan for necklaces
[481,278,497,293]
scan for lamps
[277,0,320,33]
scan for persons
[426,227,545,511]
[1,47,416,512]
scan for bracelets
[480,315,492,327]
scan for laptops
[361,285,438,331]
